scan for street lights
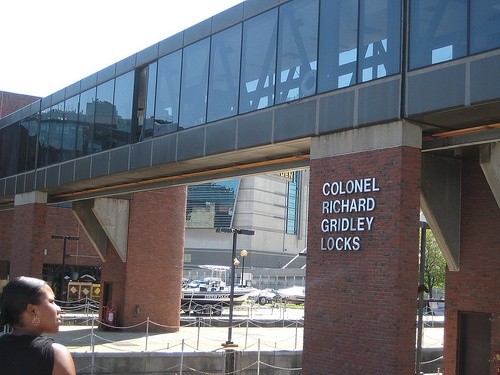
[215,228,255,347]
[238,249,247,285]
[413,221,436,375]
[51,234,81,301]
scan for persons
[0,275,77,375]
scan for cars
[182,278,305,316]
